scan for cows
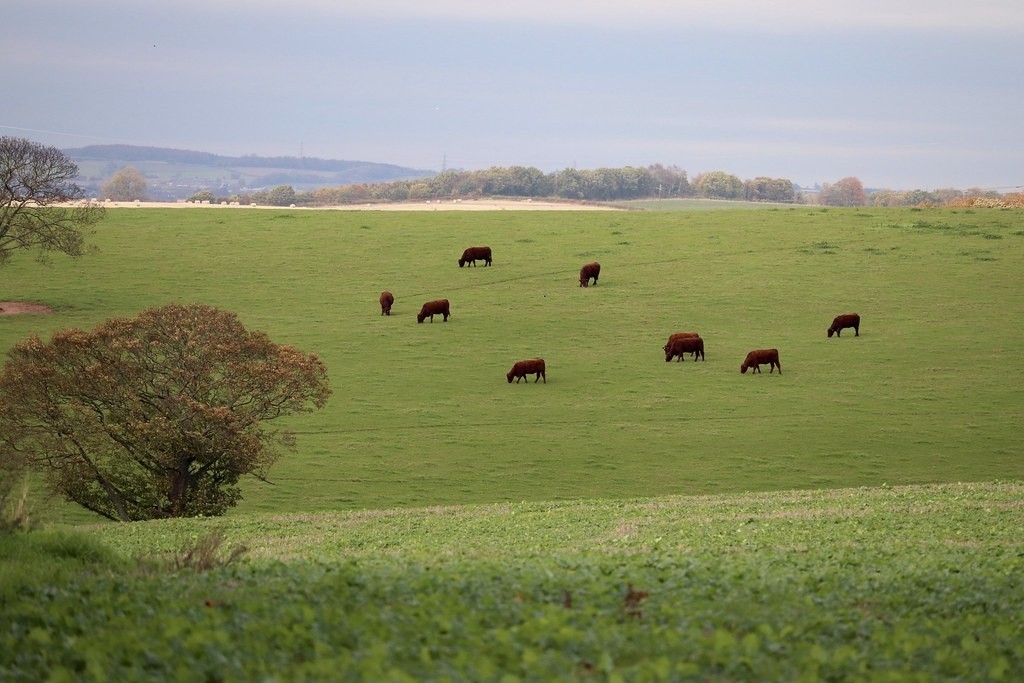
[827,313,861,338]
[458,246,493,268]
[740,348,782,375]
[578,261,601,288]
[417,298,451,323]
[506,357,546,385]
[662,332,705,363]
[379,291,395,316]
